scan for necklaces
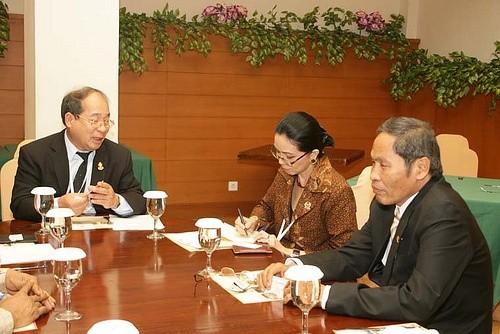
[296,175,305,188]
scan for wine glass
[290,280,321,334]
[196,276,219,333]
[54,318,82,334]
[197,228,221,277]
[143,238,164,283]
[34,195,54,233]
[146,199,166,238]
[48,218,72,248]
[53,260,82,318]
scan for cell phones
[232,243,273,255]
[0,234,38,243]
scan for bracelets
[251,216,258,231]
[114,195,121,209]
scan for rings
[267,234,270,239]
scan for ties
[69,151,93,193]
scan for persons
[235,111,359,257]
[253,117,493,334]
[10,87,146,220]
[0,267,56,334]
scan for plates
[31,187,56,195]
[143,191,168,199]
[284,265,324,281]
[45,208,75,218]
[50,247,86,261]
[195,218,224,228]
[87,319,139,334]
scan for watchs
[291,248,300,258]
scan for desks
[444,175,500,311]
[0,218,444,334]
[0,144,156,191]
[238,143,365,180]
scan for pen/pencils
[236,208,250,236]
[85,181,105,195]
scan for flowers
[201,3,249,23]
[354,10,387,35]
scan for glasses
[76,115,115,128]
[267,145,311,167]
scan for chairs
[436,134,478,178]
[0,139,38,222]
